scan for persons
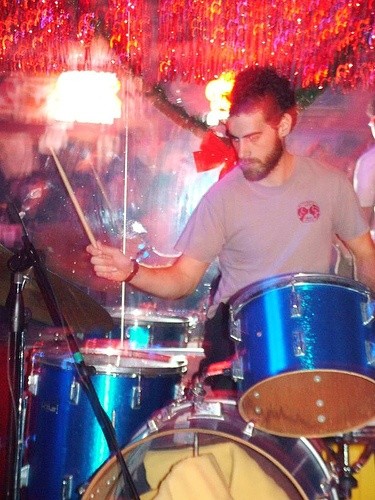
[85,64,375,390]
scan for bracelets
[120,259,140,284]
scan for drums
[25,346,188,500]
[225,272,375,438]
[83,306,191,348]
[81,398,339,500]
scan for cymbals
[0,244,113,331]
